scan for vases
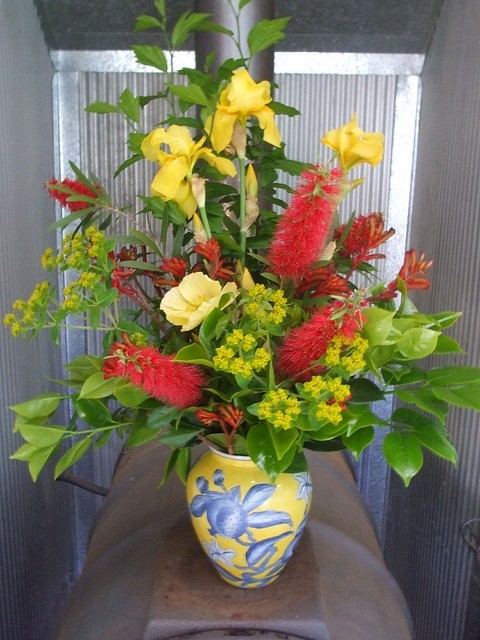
[182,433,316,592]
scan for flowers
[0,0,480,492]
